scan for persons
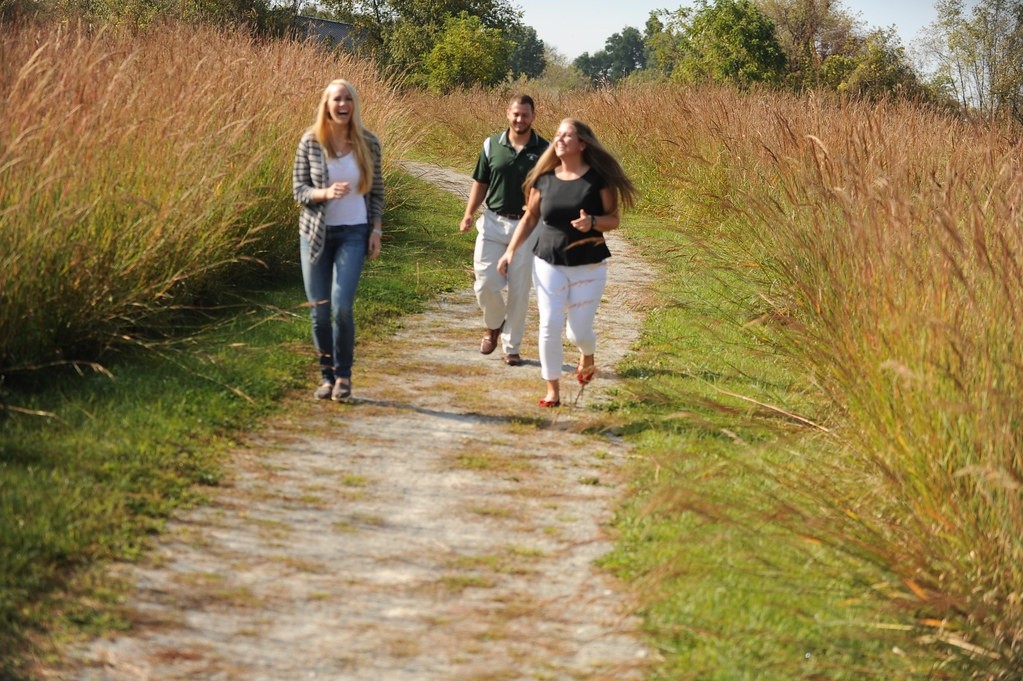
[498,118,636,407]
[460,93,552,365]
[292,78,384,402]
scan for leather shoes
[480,319,506,355]
[504,354,521,366]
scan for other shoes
[316,383,333,401]
[577,354,595,386]
[538,398,561,408]
[331,379,353,402]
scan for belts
[496,213,523,220]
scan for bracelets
[373,229,382,236]
[591,215,595,229]
